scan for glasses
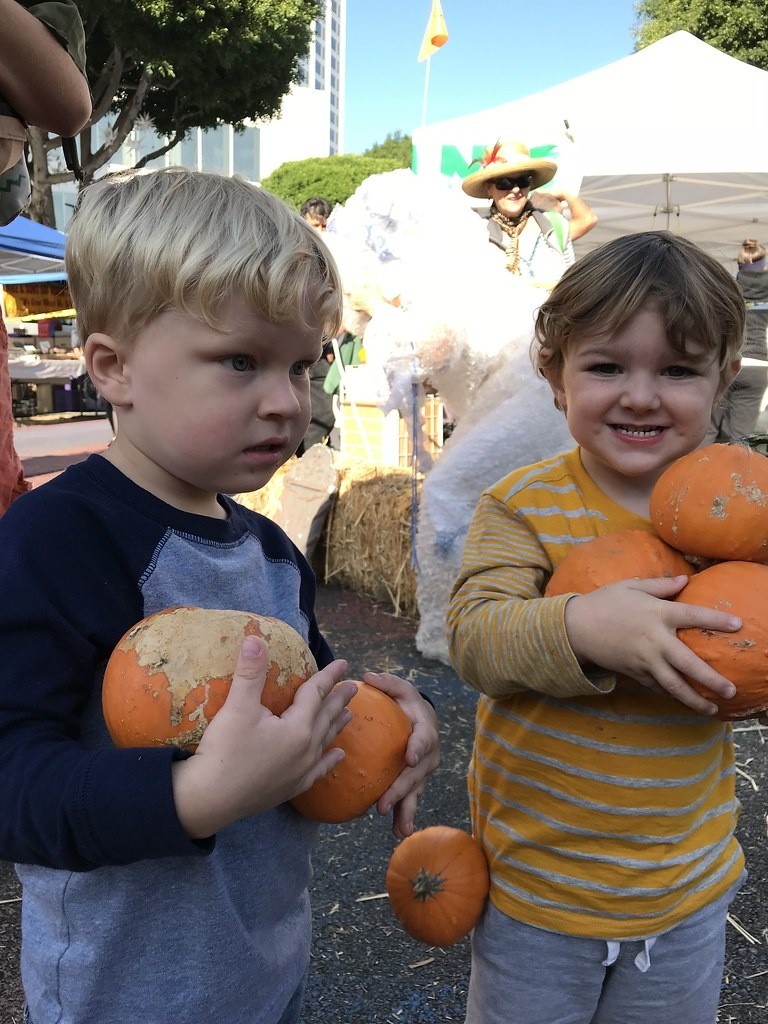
[489,175,532,190]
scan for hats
[462,141,558,199]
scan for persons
[0,166,442,1024]
[294,139,599,466]
[445,231,768,1024]
[703,238,768,458]
[0,0,94,520]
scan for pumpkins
[102,604,321,754]
[543,431,768,720]
[288,680,414,822]
[386,823,489,946]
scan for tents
[411,30,768,280]
[0,216,69,318]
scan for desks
[9,357,99,419]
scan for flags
[418,0,449,63]
